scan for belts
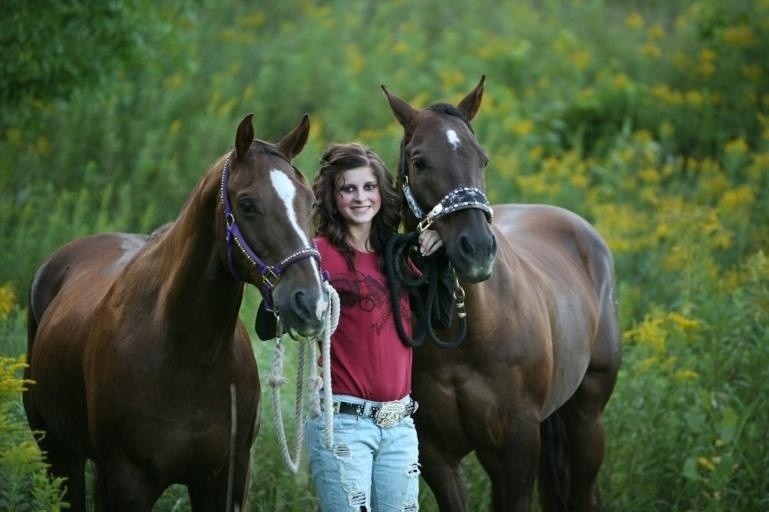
[321,399,420,419]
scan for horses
[20,111,331,512]
[378,72,624,512]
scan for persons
[255,144,454,512]
